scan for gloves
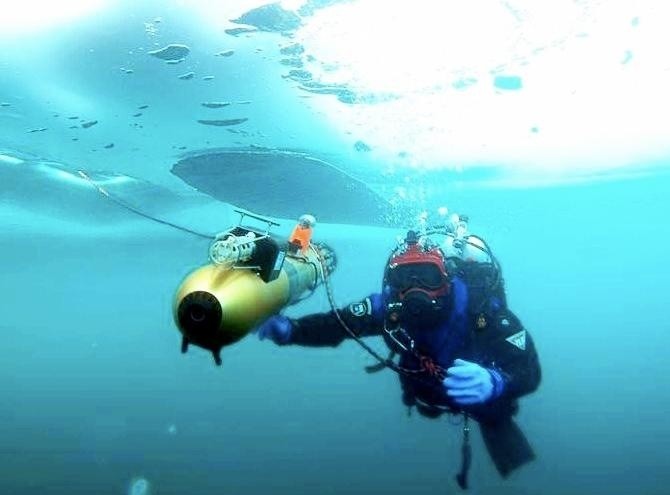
[443,359,495,406]
[258,314,291,346]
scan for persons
[256,229,544,435]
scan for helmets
[385,235,450,325]
